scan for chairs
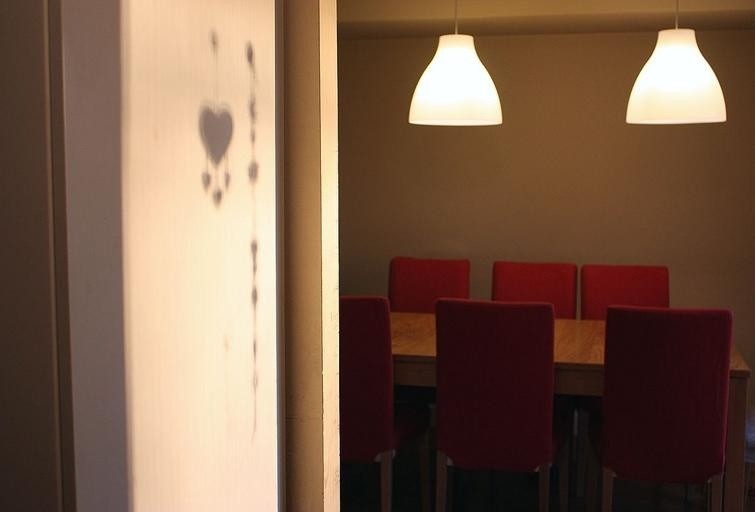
[343,290,431,510]
[385,254,472,312]
[575,262,675,321]
[592,305,733,510]
[488,259,580,321]
[434,300,573,512]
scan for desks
[389,312,755,512]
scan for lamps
[409,0,506,128]
[625,0,728,127]
